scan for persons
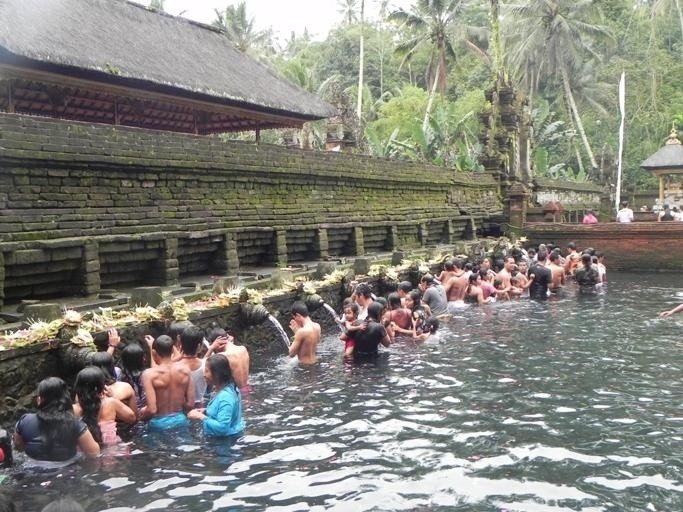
[339,241,607,354]
[583,211,598,223]
[1,326,250,468]
[658,204,683,221]
[288,300,321,364]
[660,304,683,317]
[616,201,634,222]
